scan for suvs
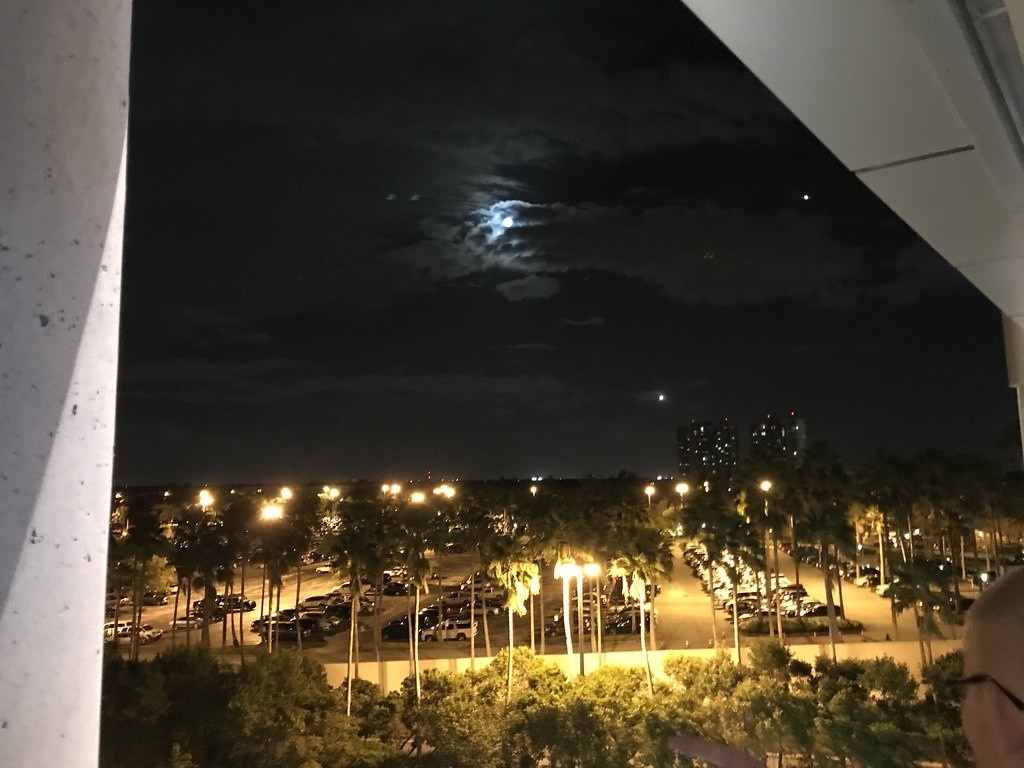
[140,592,168,606]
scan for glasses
[944,672,1024,712]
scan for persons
[960,566,1024,767]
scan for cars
[169,592,256,631]
[104,620,163,646]
[381,569,506,643]
[316,564,330,574]
[251,566,437,641]
[779,527,1023,614]
[683,540,845,631]
[545,543,661,637]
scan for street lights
[677,484,688,508]
[644,485,654,508]
